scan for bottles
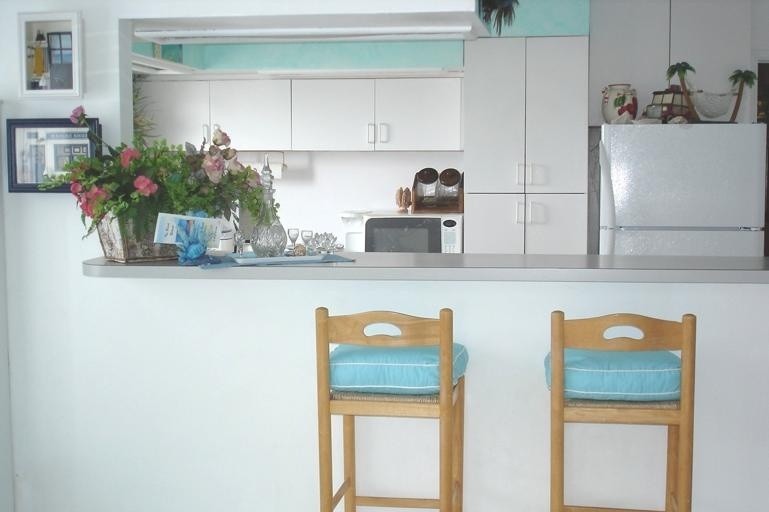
[250,154,287,255]
[437,168,461,207]
[600,84,637,124]
[415,168,441,209]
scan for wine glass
[286,229,313,255]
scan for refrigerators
[586,122,765,262]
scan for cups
[233,231,246,255]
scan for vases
[598,80,642,124]
[89,200,182,265]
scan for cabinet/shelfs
[133,70,293,153]
[461,32,590,194]
[292,71,465,153]
[461,194,591,256]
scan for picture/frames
[15,9,82,100]
[5,116,103,195]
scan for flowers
[37,100,281,243]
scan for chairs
[309,300,472,512]
[539,305,701,512]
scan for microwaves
[362,211,464,253]
